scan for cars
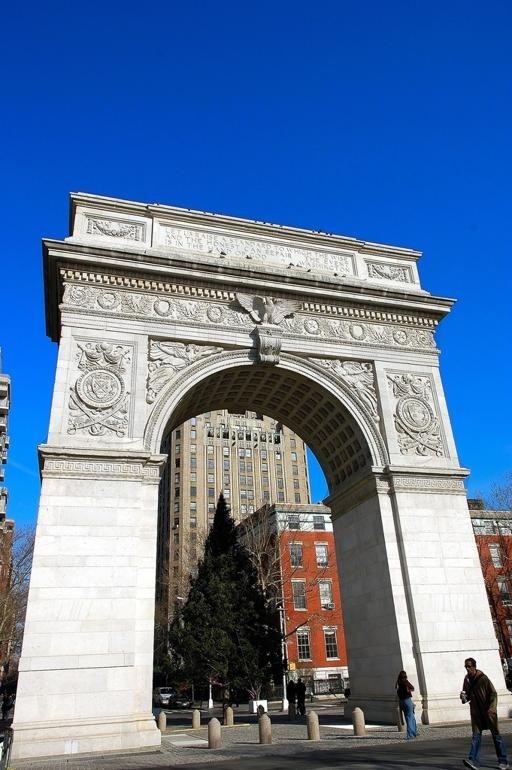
[169,694,191,708]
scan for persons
[333,360,378,417]
[295,678,306,716]
[229,689,239,707]
[398,670,419,739]
[147,343,225,404]
[287,680,297,720]
[460,658,509,770]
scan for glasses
[465,664,473,668]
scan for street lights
[175,595,216,709]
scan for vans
[153,687,174,706]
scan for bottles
[461,691,466,704]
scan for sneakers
[496,763,509,770]
[463,758,480,770]
